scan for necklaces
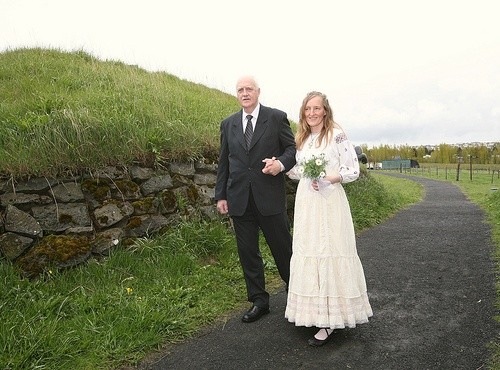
[308,132,322,149]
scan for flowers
[293,152,329,183]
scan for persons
[262,89,361,348]
[214,72,299,322]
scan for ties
[244,115,254,150]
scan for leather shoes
[308,329,337,348]
[241,304,269,322]
